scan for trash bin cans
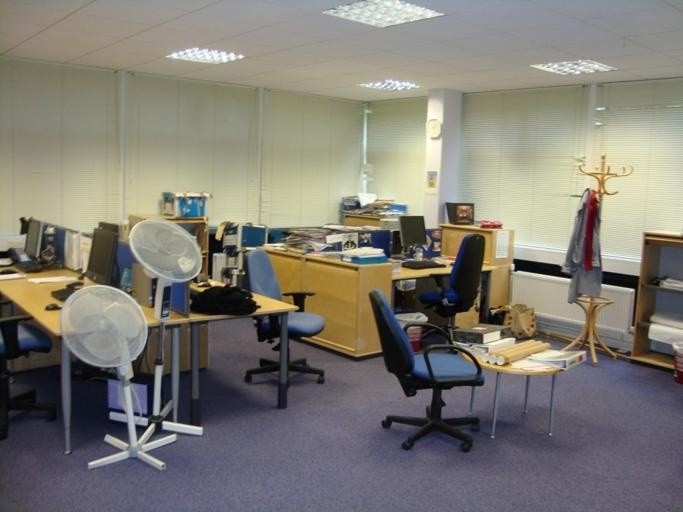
[394,313,429,354]
[672,341,683,384]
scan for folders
[527,349,587,371]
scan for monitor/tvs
[85,228,118,285]
[392,216,427,259]
[24,220,43,258]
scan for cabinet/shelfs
[439,223,515,322]
[256,246,392,360]
[127,212,209,282]
[630,231,683,371]
[343,214,381,228]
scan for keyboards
[51,287,75,303]
[15,261,43,273]
[401,261,446,269]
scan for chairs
[418,233,485,347]
[1,300,57,440]
[368,289,484,452]
[242,250,325,390]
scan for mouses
[45,303,61,310]
[0,269,15,274]
[197,281,211,287]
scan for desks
[462,350,564,439]
[0,258,189,455]
[392,262,498,328]
[190,279,300,426]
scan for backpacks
[189,284,261,316]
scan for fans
[109,219,204,446]
[60,284,177,471]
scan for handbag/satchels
[488,302,538,338]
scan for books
[453,322,586,371]
[342,192,408,218]
[282,224,388,264]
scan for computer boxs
[395,279,417,313]
[105,373,164,427]
